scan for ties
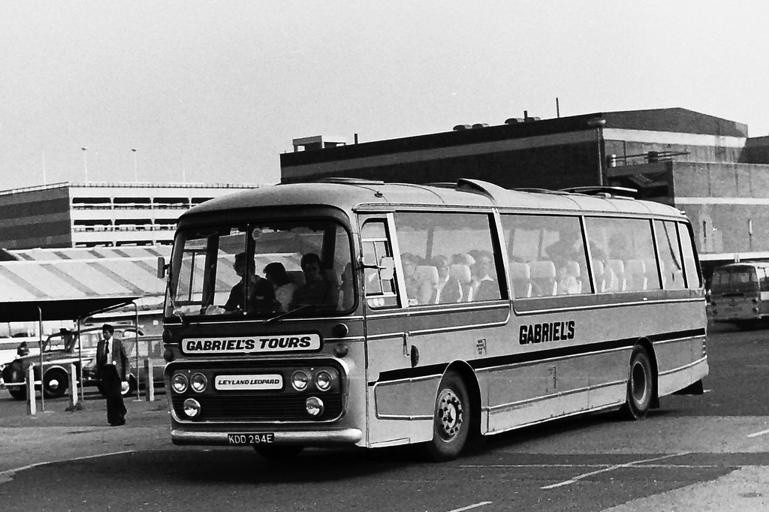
[106,340,108,353]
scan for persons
[223,251,338,318]
[92,322,133,426]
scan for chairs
[288,270,345,312]
[404,258,684,304]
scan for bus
[157,175,709,463]
[712,260,768,320]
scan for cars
[0,322,146,400]
[82,335,168,394]
